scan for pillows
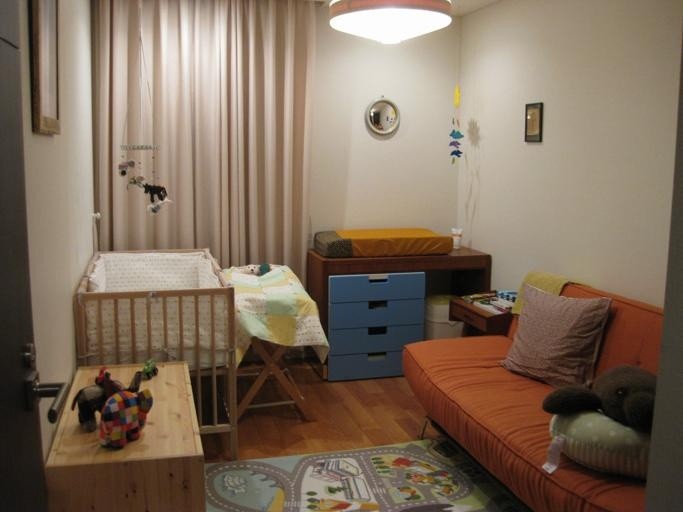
[499,285,612,389]
[548,409,650,479]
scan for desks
[307,247,492,380]
[448,288,512,338]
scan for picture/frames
[30,0,62,137]
[524,102,543,143]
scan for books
[462,290,518,316]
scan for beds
[70,249,237,463]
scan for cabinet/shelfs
[44,359,206,512]
[327,271,426,382]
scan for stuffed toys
[71,367,127,433]
[119,143,167,217]
[240,263,259,274]
[256,264,270,275]
[127,359,161,392]
[97,389,153,450]
[539,363,655,432]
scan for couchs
[402,278,668,508]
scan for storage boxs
[425,294,464,340]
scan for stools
[223,263,317,423]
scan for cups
[451,228,464,248]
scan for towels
[510,271,586,317]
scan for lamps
[327,0,454,44]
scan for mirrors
[366,96,399,135]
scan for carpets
[203,438,536,512]
